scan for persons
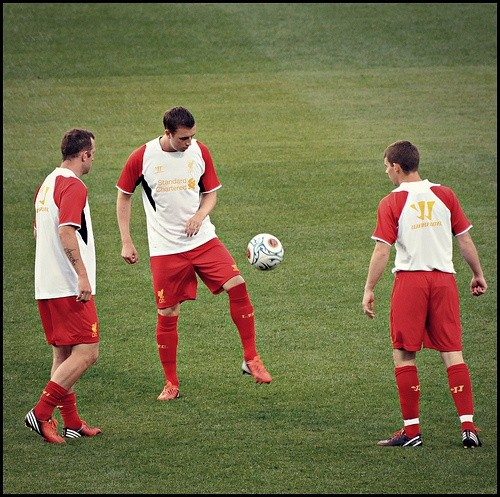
[362,140,488,449]
[23,128,102,445]
[116,107,271,400]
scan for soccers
[244,232,286,272]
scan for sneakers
[157,379,180,401]
[23,408,67,444]
[61,419,102,440]
[461,429,482,449]
[377,427,423,447]
[241,355,272,385]
